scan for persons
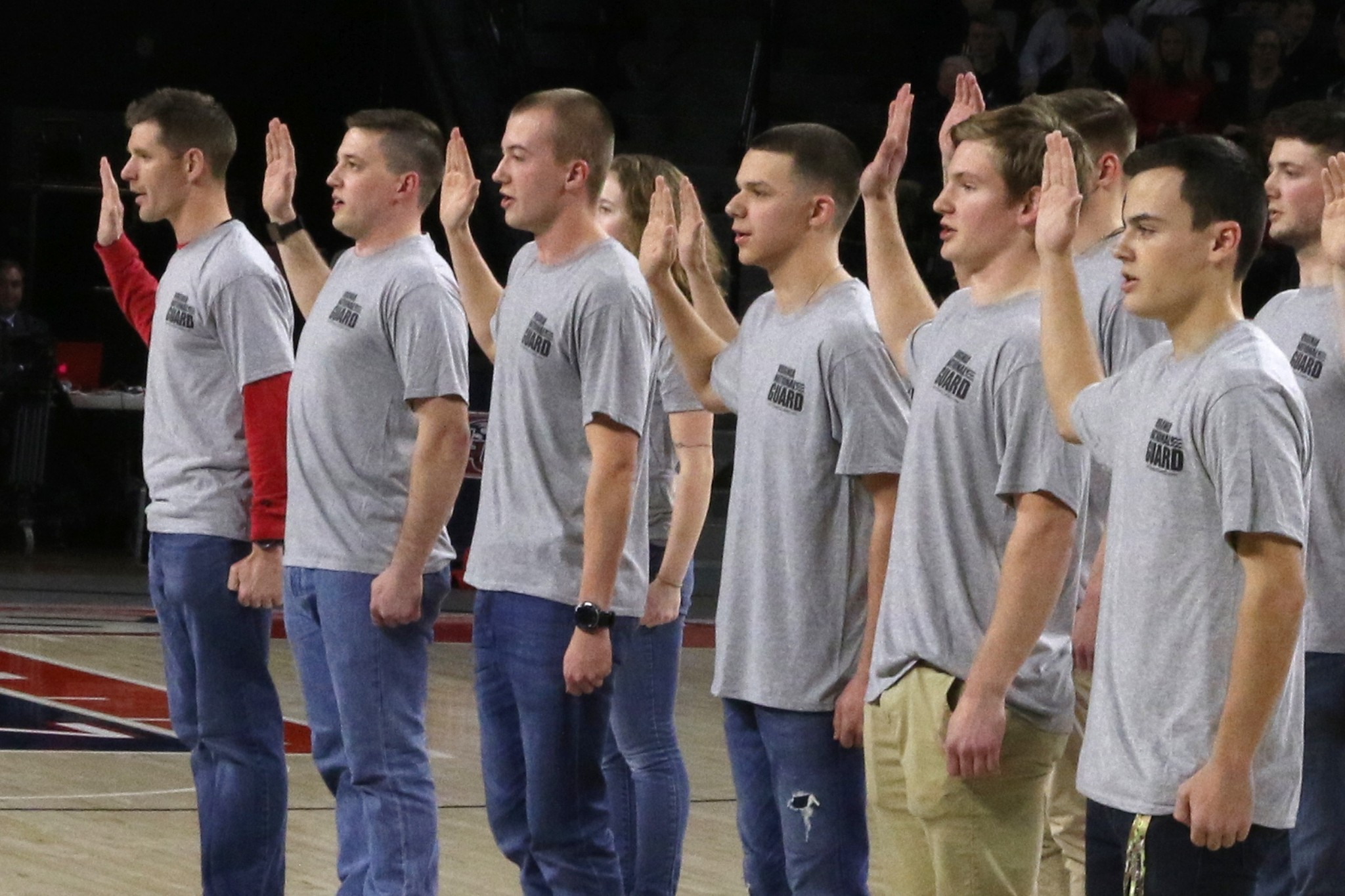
[1252,105,1345,896]
[263,108,470,896]
[598,153,726,896]
[849,83,1090,896]
[441,88,660,896]
[638,123,915,896]
[940,72,1172,896]
[1035,129,1315,896]
[93,88,294,896]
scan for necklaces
[804,265,843,308]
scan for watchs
[255,539,277,551]
[266,216,302,241]
[574,601,616,632]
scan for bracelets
[658,575,682,588]
